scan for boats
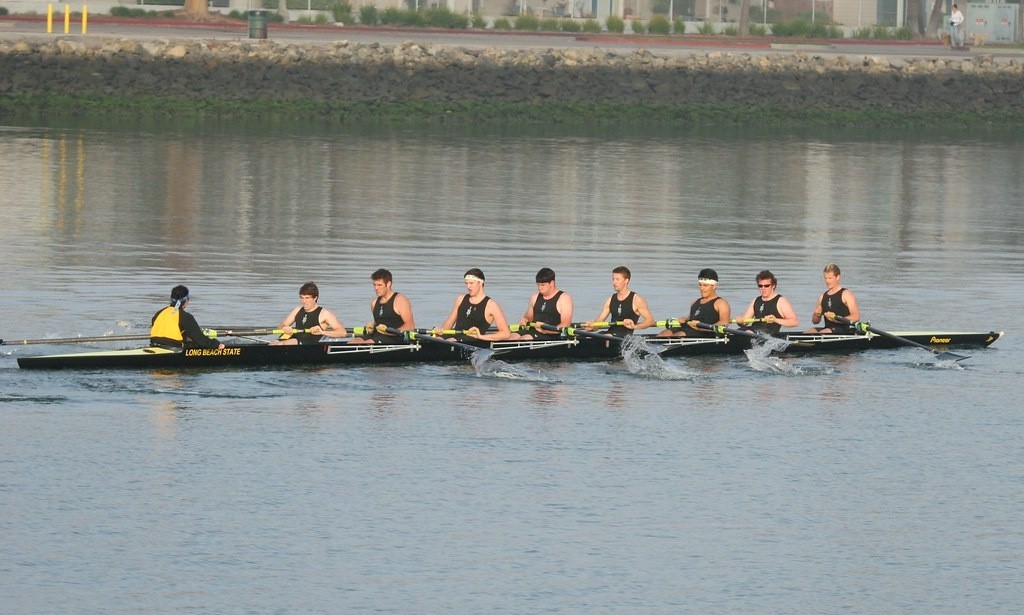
[18,330,1001,369]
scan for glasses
[758,284,771,288]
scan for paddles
[0,329,319,346]
[115,320,476,334]
[818,304,972,363]
[367,325,512,355]
[526,322,682,354]
[685,320,821,348]
[430,317,775,337]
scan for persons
[149,285,226,350]
[268,282,347,345]
[432,267,511,342]
[581,266,654,339]
[948,4,964,47]
[347,269,415,345]
[734,270,798,337]
[656,268,730,338]
[507,267,574,341]
[805,264,860,335]
